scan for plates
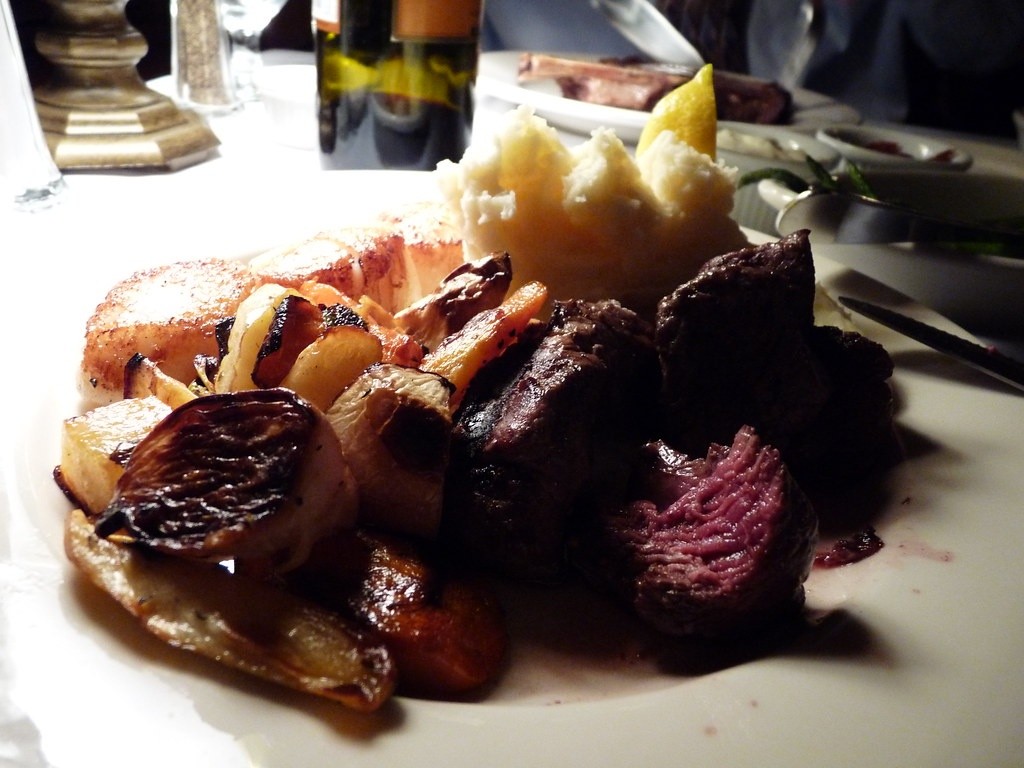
[0,354,1024,767]
[476,50,861,140]
[816,124,973,173]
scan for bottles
[311,0,481,172]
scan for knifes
[839,297,1024,392]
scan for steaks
[521,52,797,124]
[449,224,901,658]
